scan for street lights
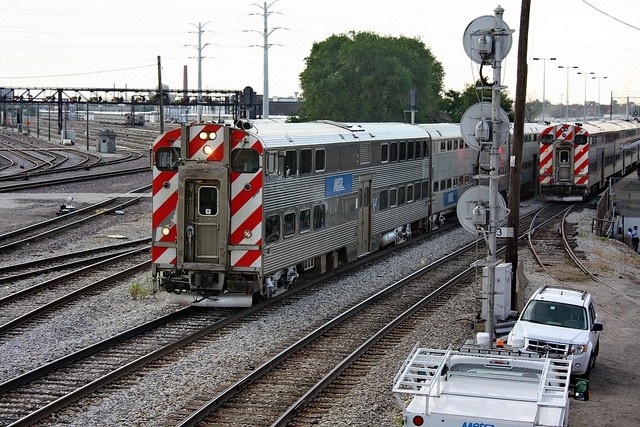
[533,57,556,118]
[241,0,290,117]
[577,71,594,120]
[558,65,577,119]
[184,21,212,123]
[590,75,606,120]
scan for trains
[538,120,638,202]
[148,117,558,308]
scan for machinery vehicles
[391,338,591,427]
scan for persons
[632,225,640,252]
[618,226,623,241]
[636,162,640,181]
[611,203,622,219]
[625,227,632,246]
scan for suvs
[508,284,603,379]
